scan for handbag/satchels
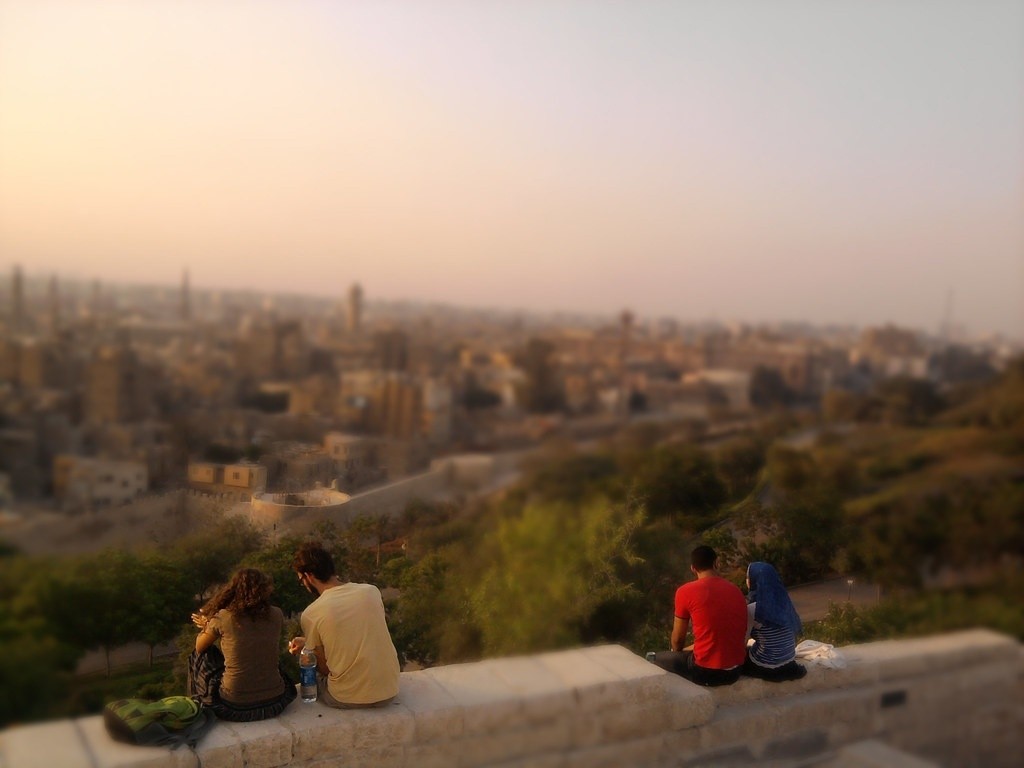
[794,640,848,669]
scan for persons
[187,568,298,723]
[745,562,803,677]
[287,542,401,709]
[647,546,748,687]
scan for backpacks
[104,695,215,750]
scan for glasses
[298,575,304,586]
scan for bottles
[299,650,317,702]
